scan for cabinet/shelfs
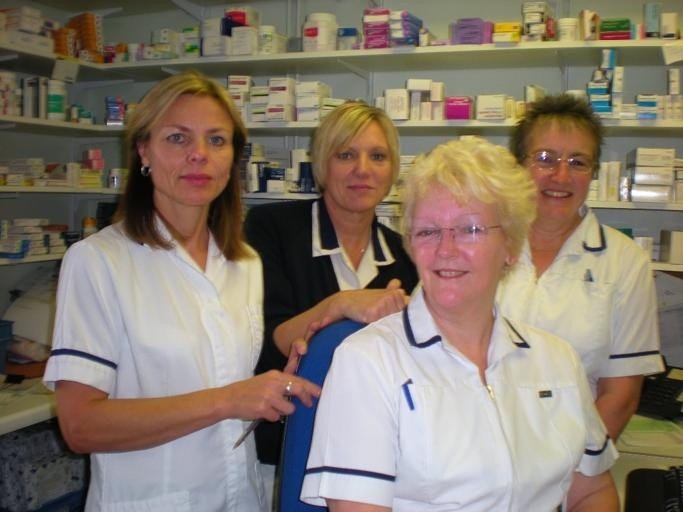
[2,3,683,78]
[1,218,682,277]
[0,81,680,138]
[2,144,681,214]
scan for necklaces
[335,230,373,257]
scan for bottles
[48,79,67,122]
[108,168,128,190]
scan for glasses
[527,153,596,174]
[412,221,501,243]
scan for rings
[284,378,299,396]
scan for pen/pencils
[584,269,593,281]
[232,419,261,452]
[403,379,414,411]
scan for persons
[504,95,670,446]
[296,134,626,511]
[249,98,419,470]
[38,66,324,512]
